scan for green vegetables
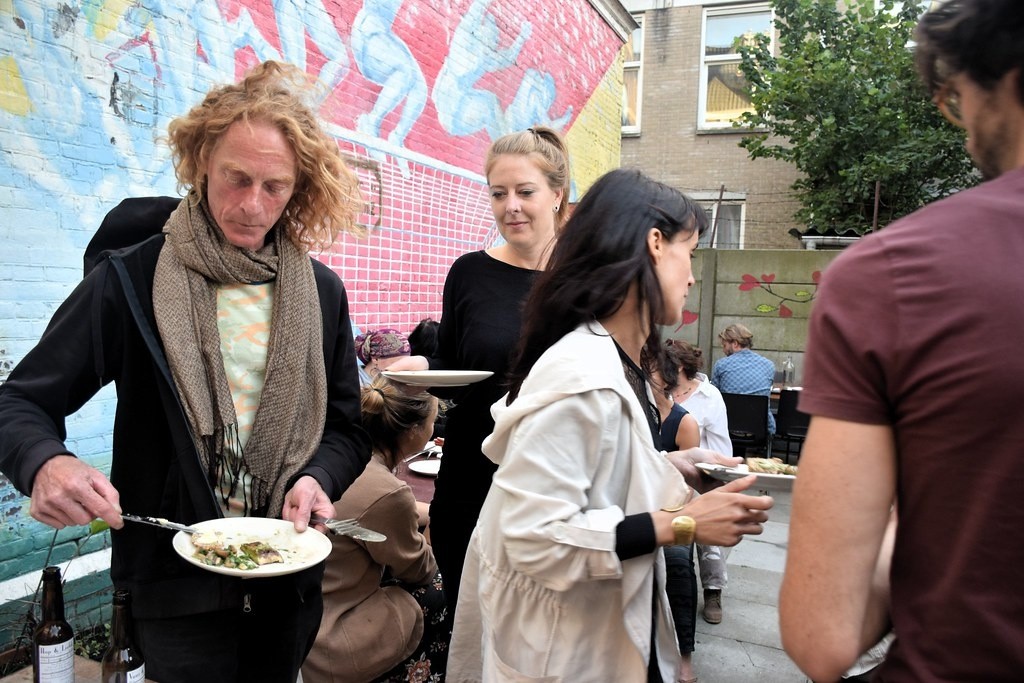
[197,546,258,569]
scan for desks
[398,453,439,503]
[771,381,802,400]
[723,388,809,461]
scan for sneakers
[703,588,724,625]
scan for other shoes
[679,656,699,683]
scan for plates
[381,369,495,387]
[694,462,801,491]
[424,440,443,453]
[172,516,333,578]
[407,460,441,476]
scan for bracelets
[661,506,696,546]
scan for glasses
[932,62,992,130]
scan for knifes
[118,513,202,536]
[311,517,387,543]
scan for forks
[310,516,362,535]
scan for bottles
[101,589,146,683]
[783,355,795,389]
[31,566,76,682]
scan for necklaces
[669,379,694,398]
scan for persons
[407,318,444,437]
[711,323,776,436]
[383,122,571,632]
[664,338,733,623]
[446,165,776,683]
[0,58,362,683]
[355,328,411,391]
[299,372,445,683]
[779,1,1024,683]
[646,348,700,683]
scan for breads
[747,457,779,475]
